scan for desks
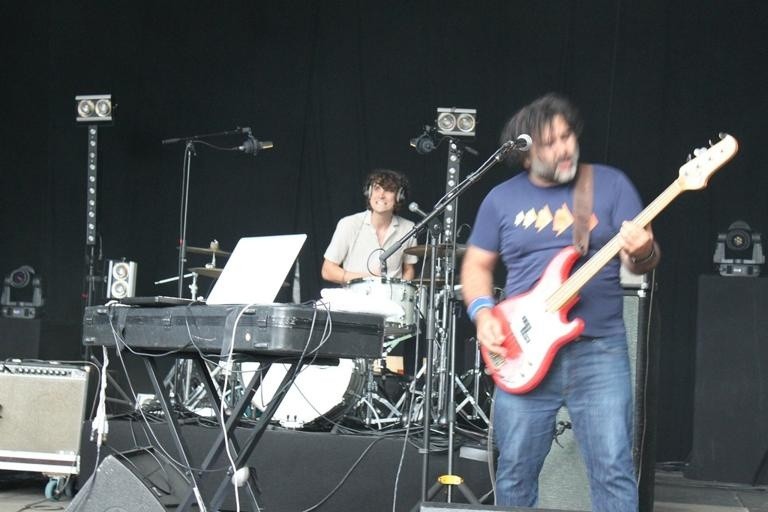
[126,348,341,512]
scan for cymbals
[404,244,468,256]
[189,267,222,279]
[411,276,450,285]
[176,246,231,257]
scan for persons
[318,168,419,283]
[458,91,664,512]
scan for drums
[238,354,369,430]
[345,277,419,336]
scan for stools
[349,319,413,428]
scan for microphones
[234,125,277,162]
[506,133,535,151]
[409,201,429,219]
[409,136,437,155]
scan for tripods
[388,225,498,511]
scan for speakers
[65,445,201,512]
[538,288,661,508]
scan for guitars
[480,131,738,395]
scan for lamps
[0,265,46,319]
[436,107,476,137]
[712,219,765,278]
[76,94,112,122]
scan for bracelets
[341,268,347,283]
[465,296,495,323]
[630,244,655,265]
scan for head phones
[361,169,407,203]
